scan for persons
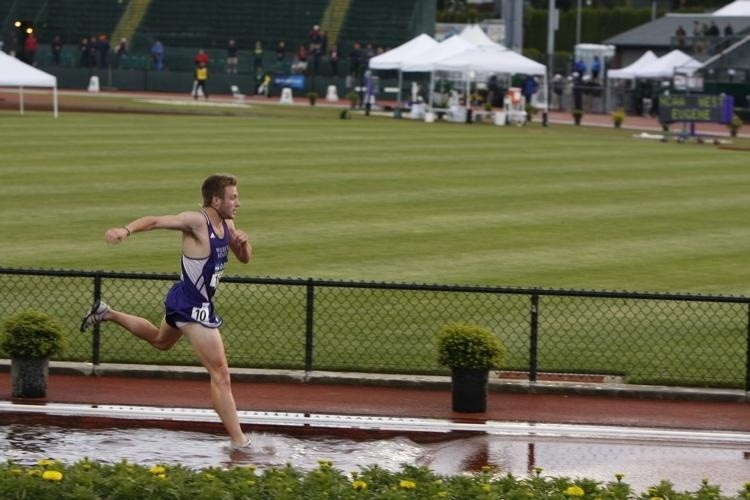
[149,40,164,71]
[78,173,265,456]
[552,56,601,111]
[328,42,386,117]
[254,25,326,98]
[226,40,238,74]
[193,50,208,101]
[3,29,37,64]
[52,36,63,66]
[676,21,733,55]
[80,35,127,67]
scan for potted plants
[308,91,358,109]
[434,322,505,413]
[572,105,742,137]
[1,310,67,397]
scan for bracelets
[121,225,131,236]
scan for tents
[1,50,57,116]
[367,23,548,112]
[606,48,704,112]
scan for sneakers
[80,300,110,334]
[230,440,277,460]
